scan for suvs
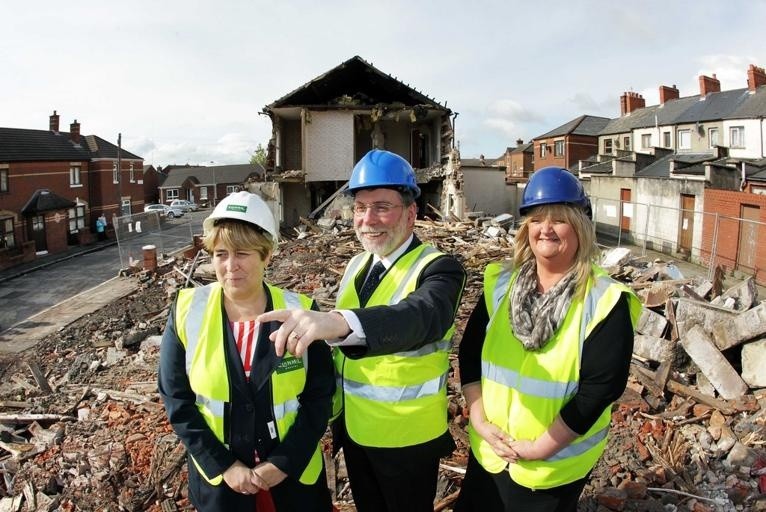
[169,200,199,212]
[143,204,184,219]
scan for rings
[240,490,248,494]
[290,331,301,341]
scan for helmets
[519,167,588,216]
[340,149,421,200]
[202,190,277,240]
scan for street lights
[210,159,218,207]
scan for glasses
[353,202,402,216]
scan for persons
[95,216,105,242]
[100,213,108,240]
[156,189,341,511]
[111,212,119,242]
[253,147,470,511]
[446,166,644,511]
